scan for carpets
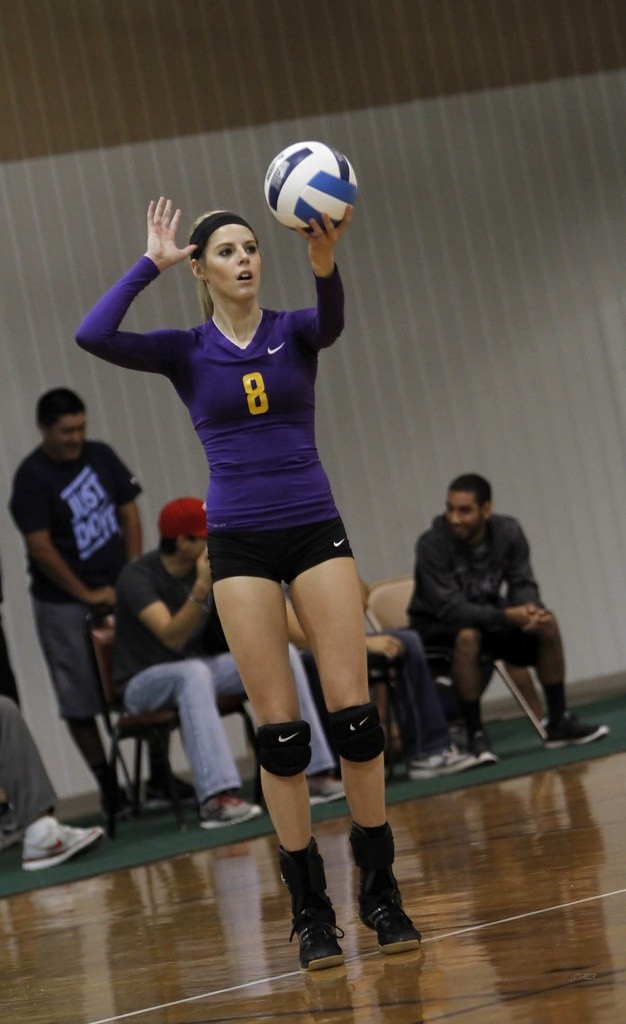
[0,689,626,898]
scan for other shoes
[100,787,133,820]
[147,775,195,803]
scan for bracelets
[190,593,204,605]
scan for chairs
[362,573,551,743]
[88,608,260,832]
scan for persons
[0,550,104,873]
[115,498,475,831]
[405,474,610,766]
[7,388,197,823]
[0,763,626,1024]
[76,195,425,971]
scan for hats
[159,498,208,538]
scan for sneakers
[300,963,356,1023]
[358,887,421,954]
[305,774,345,806]
[289,907,344,970]
[375,948,425,1018]
[543,713,609,749]
[21,823,104,870]
[407,746,475,780]
[471,729,498,766]
[198,794,262,829]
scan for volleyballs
[264,140,357,234]
